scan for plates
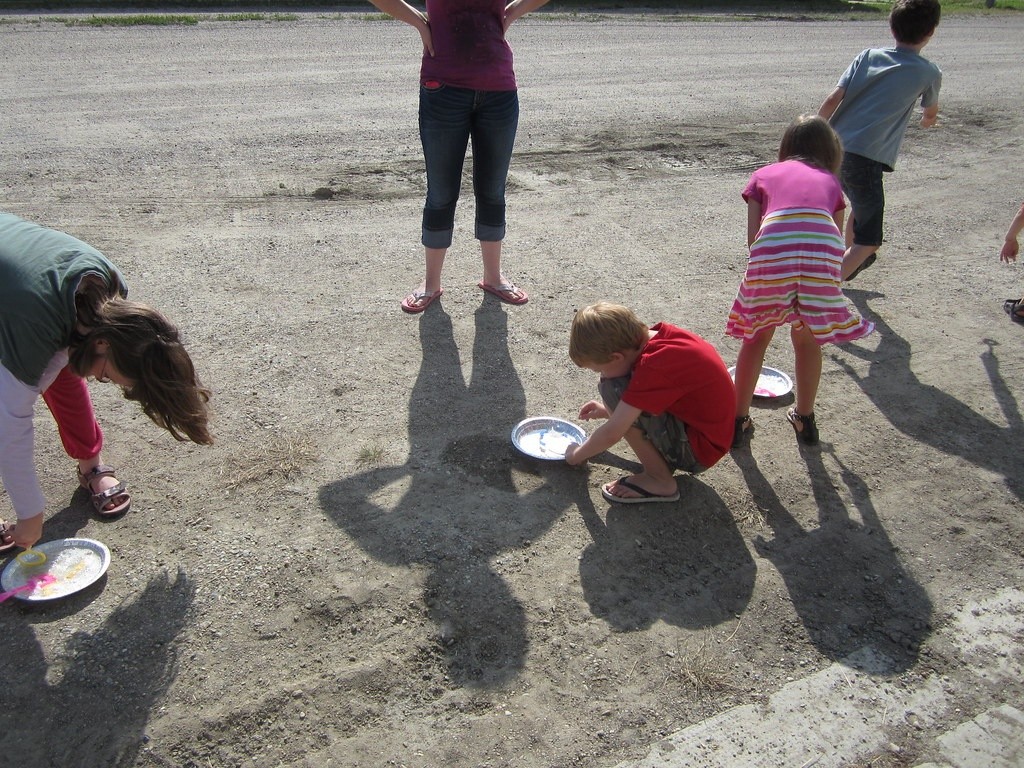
[1,538,111,602]
[511,416,588,461]
[727,365,794,399]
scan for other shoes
[845,252,877,281]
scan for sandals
[1004,299,1024,322]
[76,464,130,516]
[733,416,753,448]
[0,524,15,551]
[786,406,818,446]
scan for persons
[566,302,738,502]
[0,213,214,552]
[817,0,943,282]
[725,114,876,442]
[1000,202,1024,322]
[371,0,550,312]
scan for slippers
[601,476,680,503]
[402,287,443,312]
[478,279,528,304]
